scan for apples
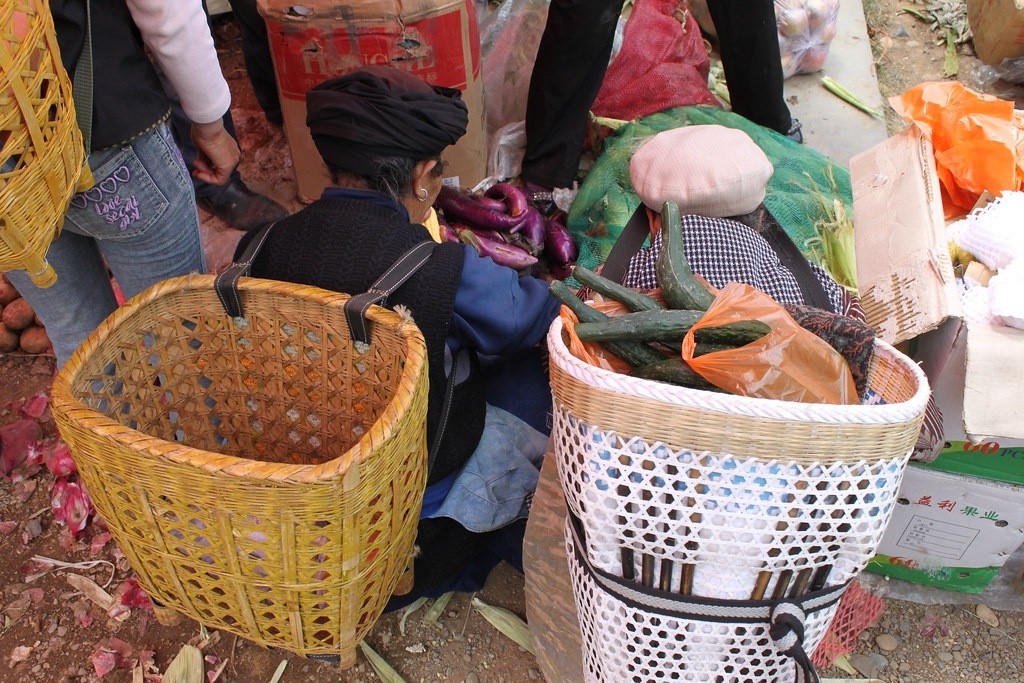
[773,0,839,75]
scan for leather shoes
[527,180,556,218]
[195,179,290,229]
[785,118,803,144]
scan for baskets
[53,274,428,670]
[2,1,95,292]
[544,303,931,683]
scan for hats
[630,123,776,218]
[306,64,469,159]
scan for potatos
[0,273,54,355]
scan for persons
[513,0,793,217]
[2,1,244,446]
[153,0,294,232]
[230,64,561,615]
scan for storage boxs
[848,120,1024,454]
[256,0,489,205]
[861,464,1024,596]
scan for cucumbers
[546,201,772,393]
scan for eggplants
[431,181,579,269]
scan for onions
[46,435,79,478]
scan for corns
[819,216,856,287]
